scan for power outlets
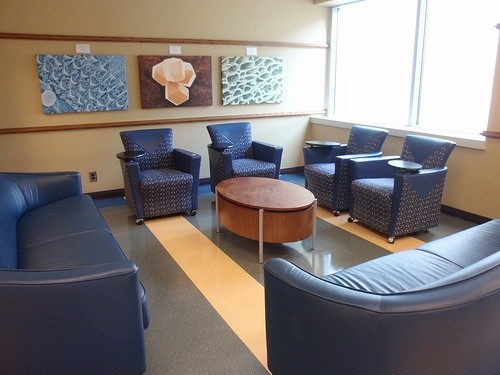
[89,172,97,182]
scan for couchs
[264,218,500,375]
[348,134,456,244]
[303,124,390,216]
[117,128,201,225]
[0,171,151,375]
[206,122,283,195]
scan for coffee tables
[215,176,317,264]
